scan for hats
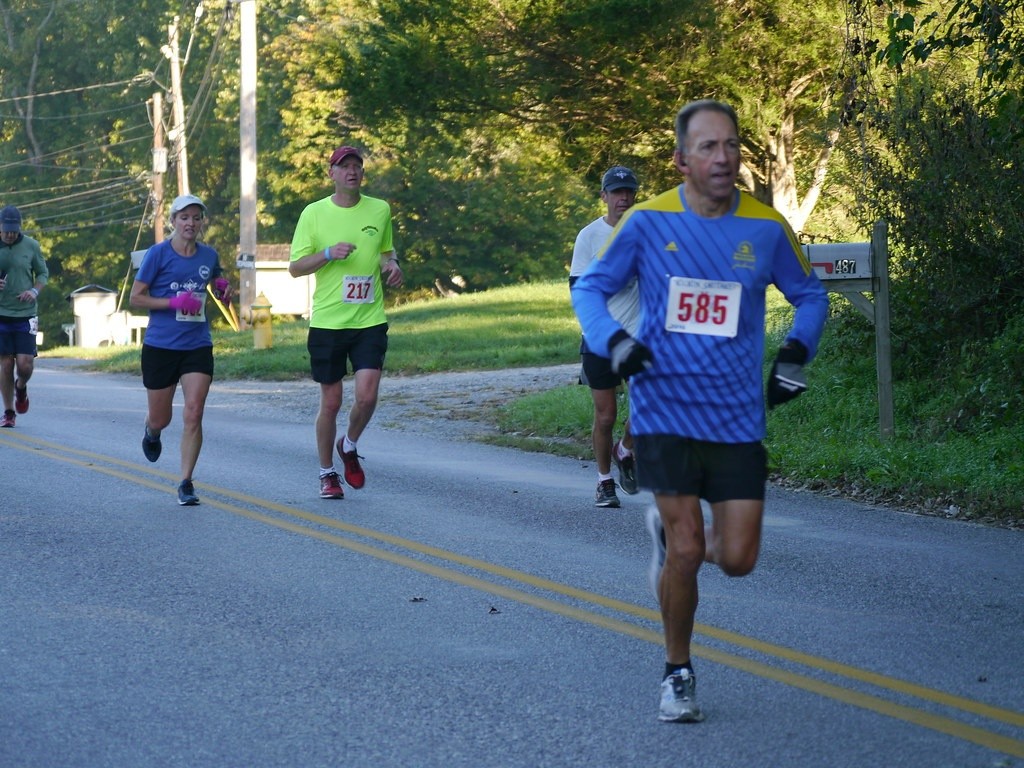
[331,146,363,166]
[601,166,638,192]
[0,206,21,232]
[169,195,207,218]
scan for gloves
[608,330,654,381]
[169,289,202,311]
[216,278,229,304]
[767,339,809,410]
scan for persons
[568,166,640,507]
[128,195,235,506]
[570,102,830,724]
[289,145,402,499]
[0,206,48,428]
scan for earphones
[679,158,687,167]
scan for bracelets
[388,258,400,267]
[32,288,38,296]
[325,247,332,260]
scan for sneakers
[645,506,669,606]
[612,441,640,495]
[177,480,199,506]
[334,435,367,490]
[15,379,29,414]
[659,668,704,723]
[0,411,16,426]
[142,426,162,463]
[594,478,620,508]
[318,472,345,500]
